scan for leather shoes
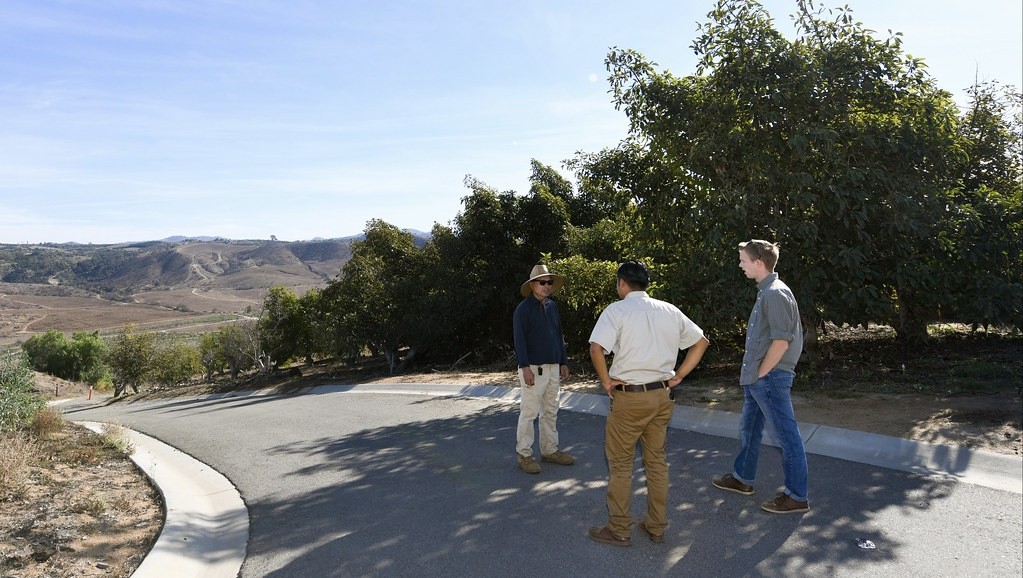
[638,519,665,542]
[590,526,631,546]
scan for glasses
[531,280,553,285]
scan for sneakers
[712,473,755,496]
[760,492,811,512]
[517,451,575,473]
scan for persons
[512,265,579,475]
[711,239,811,514]
[585,262,708,545]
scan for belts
[613,380,669,390]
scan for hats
[520,265,563,299]
